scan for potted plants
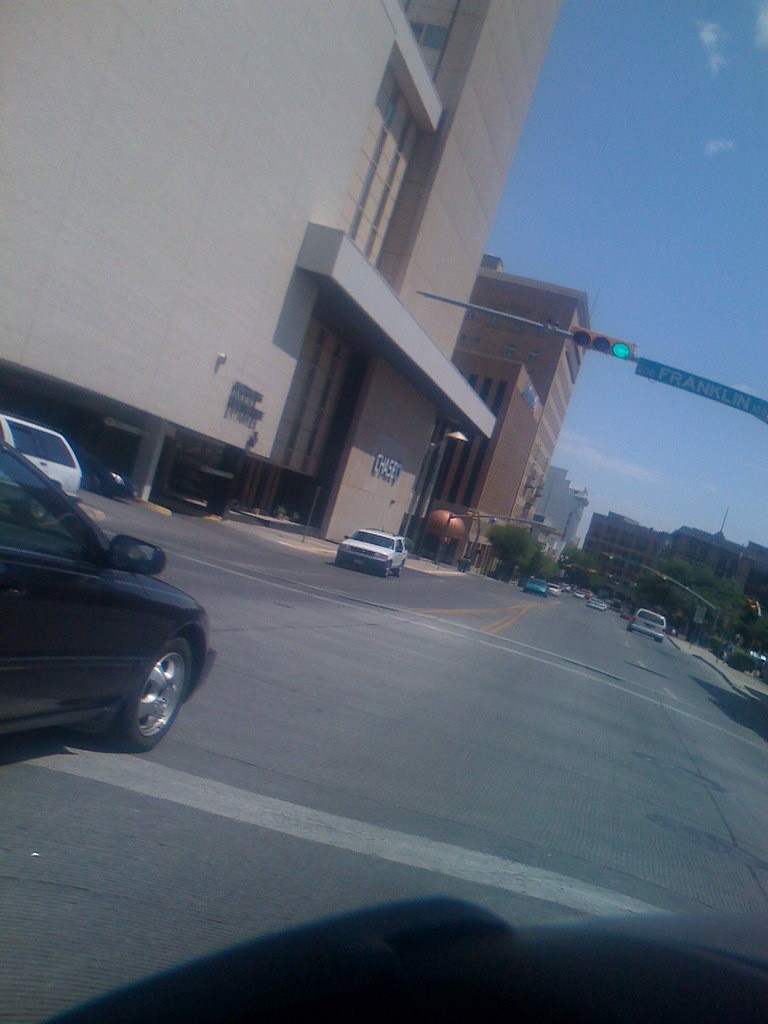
[272,504,289,520]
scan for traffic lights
[571,326,637,362]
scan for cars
[334,530,411,579]
[627,608,667,643]
[523,579,621,611]
[0,441,212,754]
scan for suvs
[0,413,84,500]
